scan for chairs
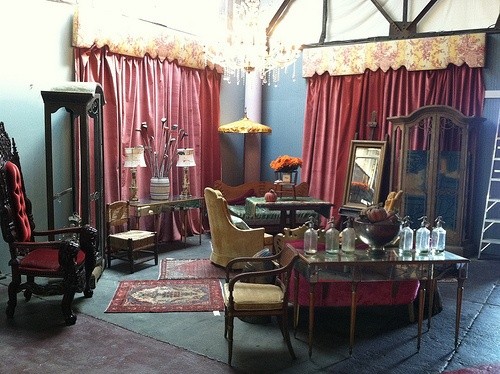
[0,121,99,325]
[223,243,299,366]
[204,187,274,269]
[106,199,157,274]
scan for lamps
[123,146,147,203]
[175,148,196,196]
[202,0,301,88]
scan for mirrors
[341,139,388,211]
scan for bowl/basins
[353,220,401,254]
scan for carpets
[156,257,238,279]
[104,279,226,313]
[1,233,348,374]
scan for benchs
[273,222,421,323]
[213,179,321,230]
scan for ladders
[478,112,500,259]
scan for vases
[282,174,292,182]
[150,177,170,200]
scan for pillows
[230,215,250,229]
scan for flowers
[269,155,303,174]
[350,182,370,193]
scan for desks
[128,194,205,260]
[245,196,335,233]
[296,247,471,357]
